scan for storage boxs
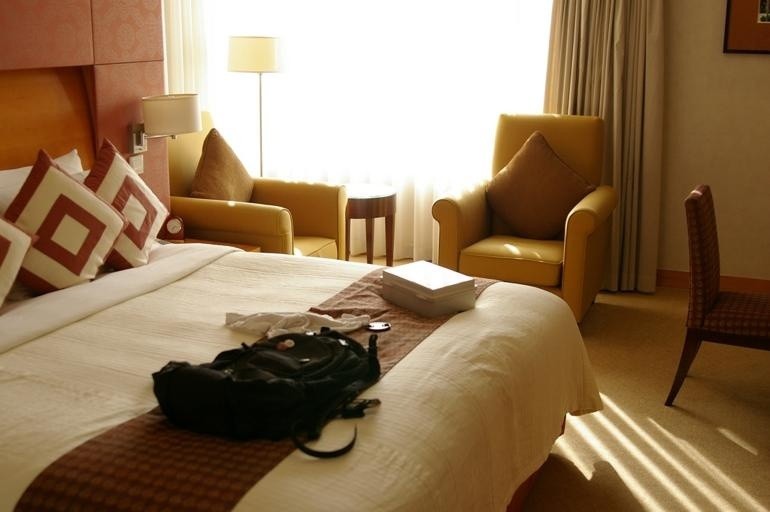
[381,260,476,319]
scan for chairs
[168,110,349,261]
[664,183,770,407]
[431,112,619,325]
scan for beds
[0,71,604,512]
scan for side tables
[345,191,397,267]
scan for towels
[224,308,373,345]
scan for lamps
[129,93,196,154]
[227,36,282,177]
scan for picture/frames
[722,0,770,54]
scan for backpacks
[152,327,379,442]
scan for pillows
[0,212,40,308]
[3,148,130,293]
[485,130,597,240]
[189,128,256,203]
[1,148,83,218]
[83,137,171,271]
